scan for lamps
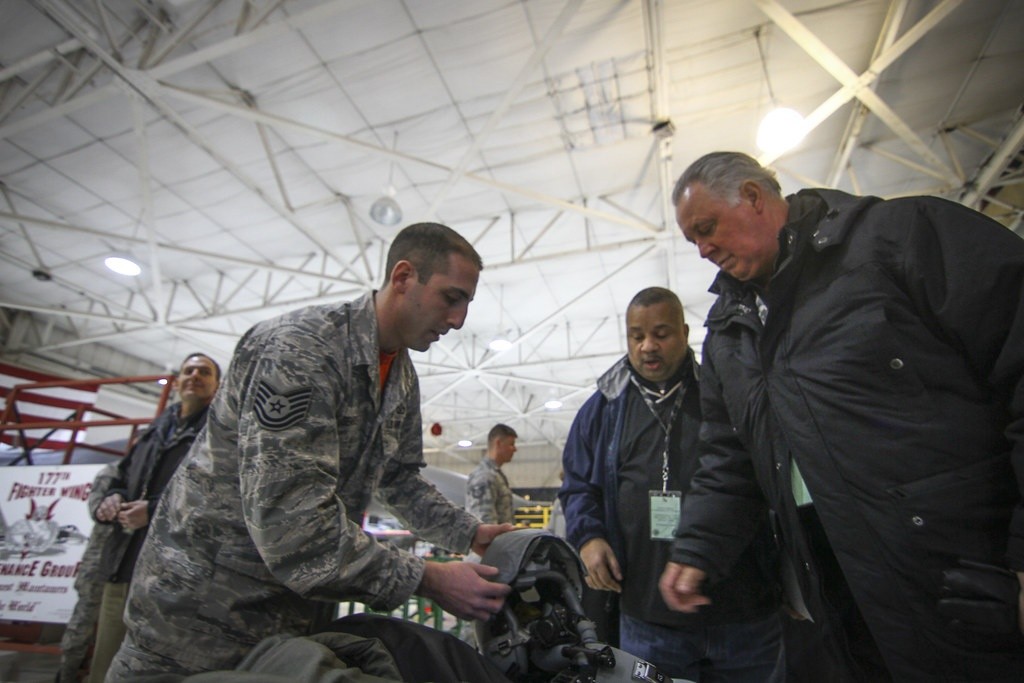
[370,133,401,223]
[105,207,147,277]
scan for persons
[658,150,1023,683]
[559,287,788,682]
[53,222,567,683]
